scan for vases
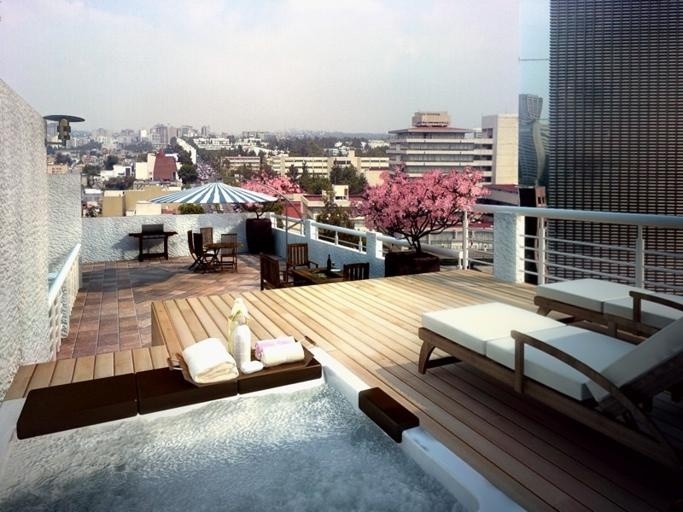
[231,327,250,373]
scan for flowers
[228,296,250,324]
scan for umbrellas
[147,182,278,241]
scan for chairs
[283,242,319,282]
[417,300,682,473]
[534,277,683,338]
[187,227,238,274]
[343,262,369,282]
[259,252,295,291]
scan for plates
[330,269,340,272]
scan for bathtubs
[0,347,519,512]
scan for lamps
[44,113,85,146]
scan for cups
[331,262,335,268]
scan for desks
[291,267,343,286]
[129,231,178,262]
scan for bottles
[326,254,331,270]
[229,318,253,368]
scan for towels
[180,325,305,385]
